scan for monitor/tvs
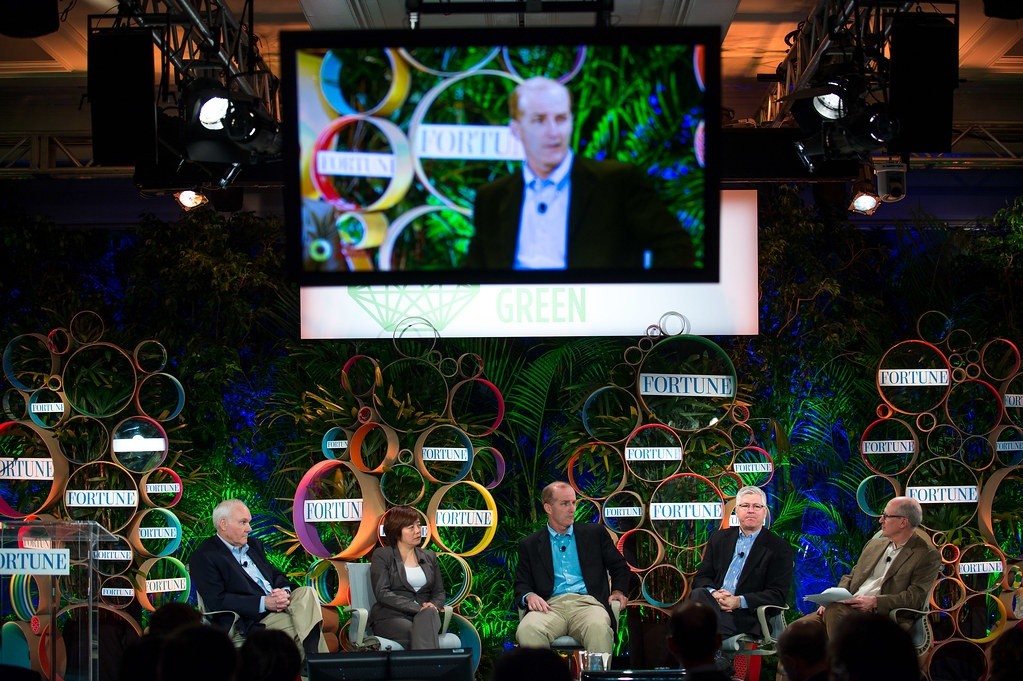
[306,646,474,681]
[278,22,721,284]
[582,668,687,681]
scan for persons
[368,506,446,651]
[776,496,942,681]
[459,76,694,267]
[514,481,630,670]
[106,600,304,681]
[490,603,920,681]
[690,486,793,640]
[189,499,331,681]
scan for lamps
[775,40,904,213]
[135,78,283,214]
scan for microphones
[886,556,891,562]
[420,559,424,563]
[538,202,547,212]
[740,552,744,558]
[562,546,565,551]
[244,561,247,567]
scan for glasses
[737,502,765,511]
[882,510,905,520]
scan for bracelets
[284,589,290,593]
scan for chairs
[889,565,941,656]
[345,562,461,651]
[197,591,244,648]
[515,571,620,651]
[718,602,790,680]
